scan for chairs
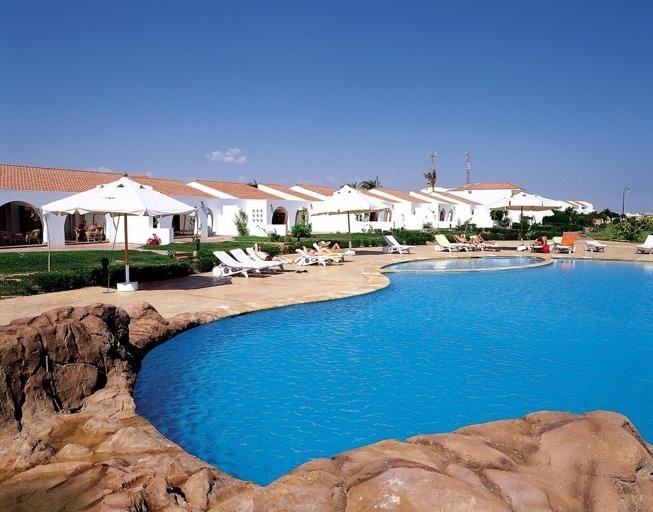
[211,231,356,285]
[529,233,607,254]
[635,234,653,255]
[434,233,497,254]
[382,235,416,255]
[74,227,105,242]
[0,230,41,245]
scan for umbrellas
[488,191,564,246]
[42,171,198,282]
[309,184,391,249]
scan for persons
[71,219,104,242]
[300,245,338,257]
[529,232,548,253]
[146,235,159,245]
[476,232,496,246]
[252,242,301,265]
[145,232,160,245]
[316,240,348,253]
[454,232,482,246]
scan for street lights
[623,187,632,214]
